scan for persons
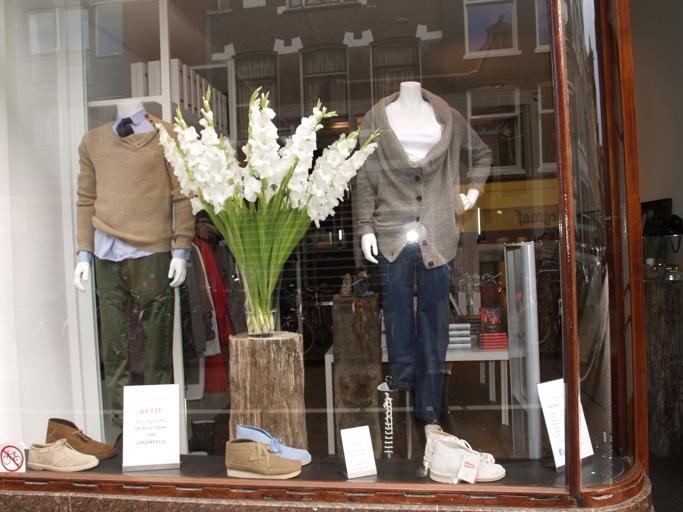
[74,96,197,430]
[355,79,493,424]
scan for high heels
[483,308,497,333]
[353,275,366,297]
[340,272,352,297]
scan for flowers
[141,84,379,333]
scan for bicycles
[279,273,378,357]
[474,267,561,344]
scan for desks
[324,342,511,456]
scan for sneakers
[45,417,118,459]
[235,423,313,466]
[225,439,302,479]
[429,438,506,485]
[422,430,496,470]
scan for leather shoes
[28,438,100,472]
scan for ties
[116,118,135,136]
